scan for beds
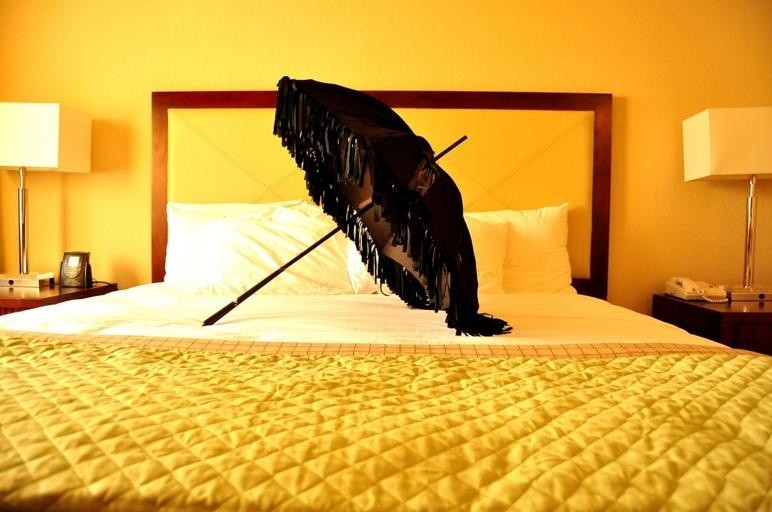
[0,88,772,508]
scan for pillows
[464,203,578,306]
[161,195,405,308]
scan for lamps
[680,105,772,304]
[0,101,94,292]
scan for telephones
[665,277,727,301]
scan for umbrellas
[201,76,513,337]
